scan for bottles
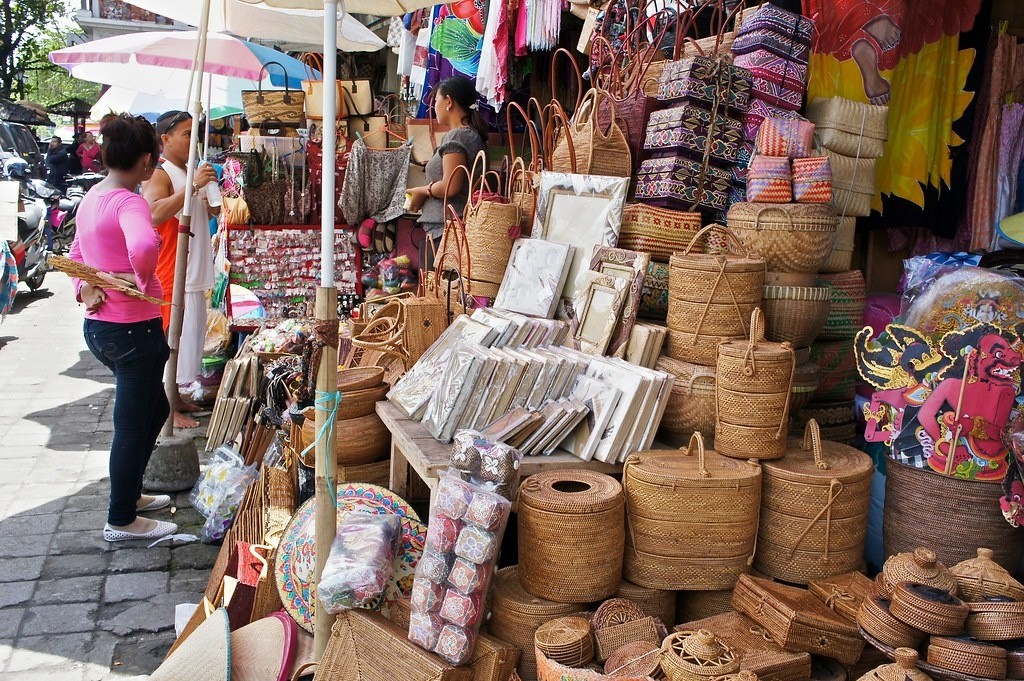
[202,161,223,208]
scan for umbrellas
[49,0,454,666]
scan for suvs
[0,122,48,182]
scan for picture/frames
[390,172,678,466]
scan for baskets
[263,289,446,542]
[291,97,1024,681]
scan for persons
[67,110,179,539]
[44,136,69,190]
[145,111,219,429]
[76,131,103,173]
[404,76,488,271]
[67,132,83,174]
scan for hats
[147,483,429,681]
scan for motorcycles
[22,178,87,254]
[0,195,54,322]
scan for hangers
[357,114,410,150]
[314,115,355,146]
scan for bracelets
[193,182,198,196]
[427,180,437,199]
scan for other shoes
[136,495,170,512]
[103,520,178,542]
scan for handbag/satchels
[405,91,452,166]
[336,50,377,117]
[241,62,306,125]
[339,86,389,149]
[422,0,768,307]
[301,52,343,119]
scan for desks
[374,401,675,523]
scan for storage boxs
[673,566,886,680]
[632,159,734,212]
[658,55,753,106]
[643,109,745,164]
[237,135,307,166]
[718,6,820,233]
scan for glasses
[165,111,192,134]
